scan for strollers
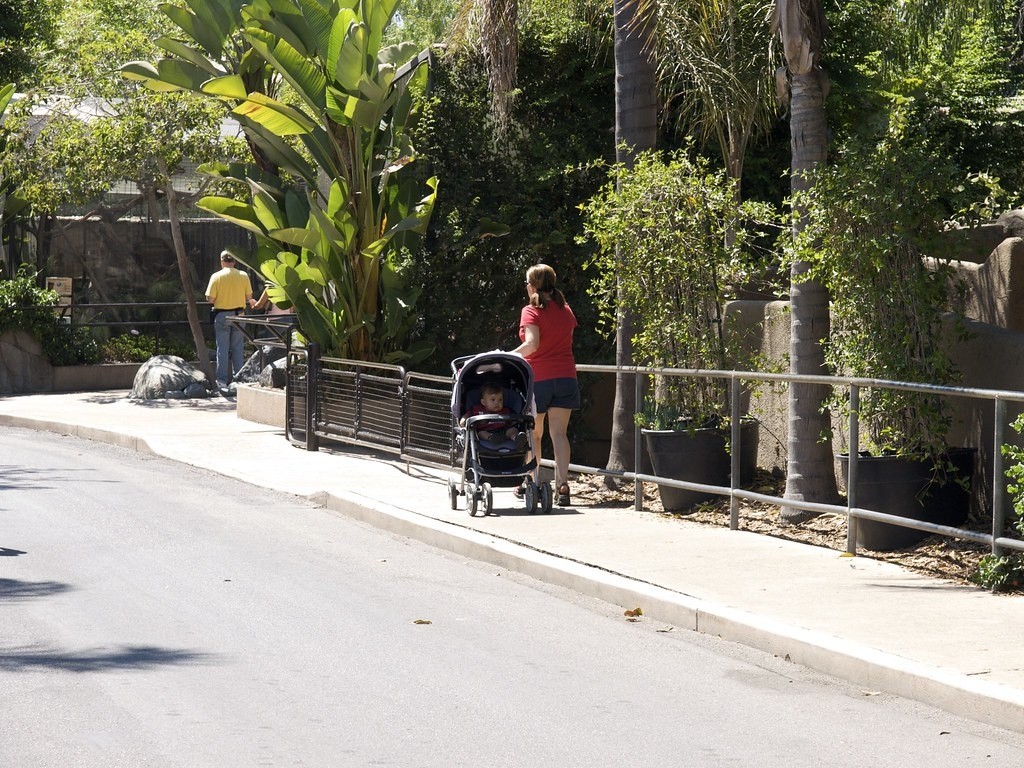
[448,350,553,517]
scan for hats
[221,250,235,262]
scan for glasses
[524,281,529,285]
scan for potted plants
[535,132,973,556]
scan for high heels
[553,483,570,506]
[514,484,526,498]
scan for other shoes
[515,432,527,452]
[489,433,506,442]
[216,380,226,388]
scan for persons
[204,250,253,389]
[249,287,295,343]
[459,382,528,449]
[507,264,581,506]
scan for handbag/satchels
[210,307,243,323]
